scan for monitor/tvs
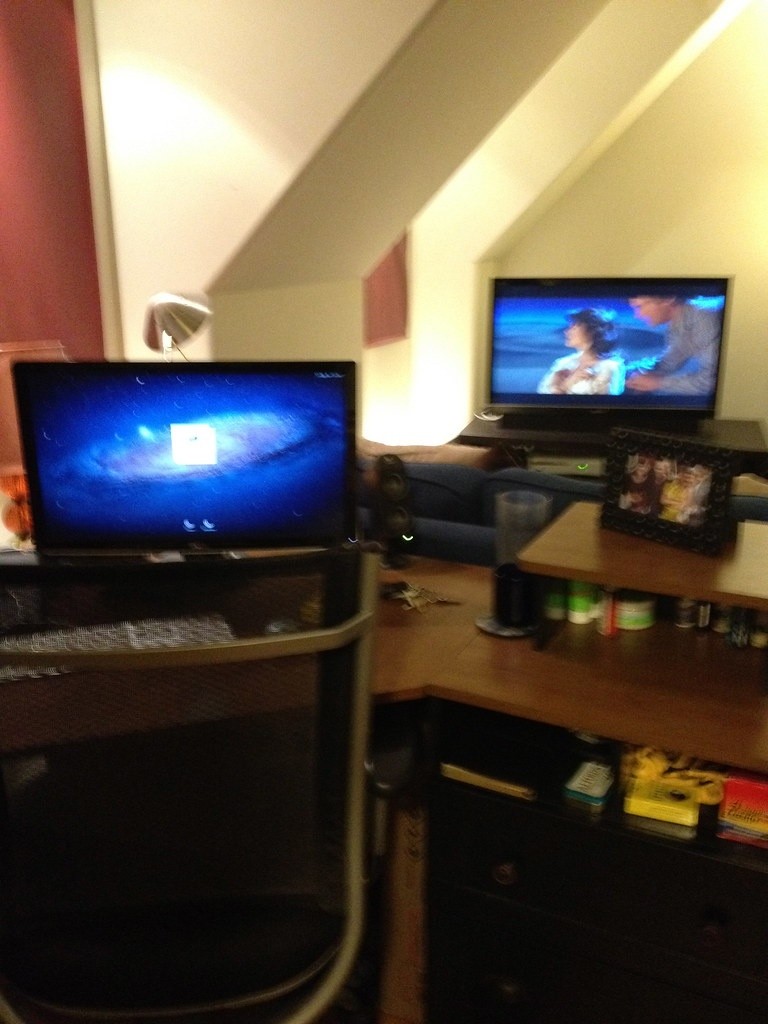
[485,277,730,434]
[9,358,355,562]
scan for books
[717,780,768,849]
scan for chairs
[0,542,370,1024]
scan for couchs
[358,437,768,571]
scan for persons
[626,297,720,395]
[536,308,626,395]
[619,454,712,524]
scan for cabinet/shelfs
[455,415,768,469]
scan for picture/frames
[601,426,736,556]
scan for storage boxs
[718,770,768,849]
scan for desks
[0,502,768,1024]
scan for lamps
[143,291,210,358]
